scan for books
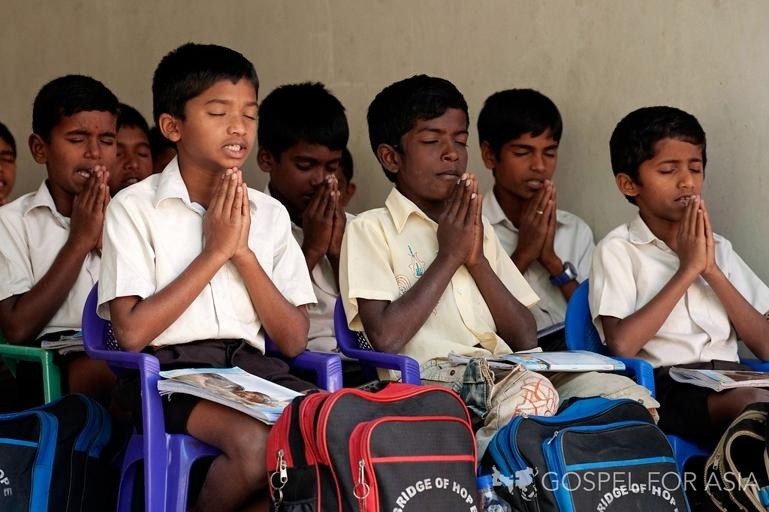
[669,367,769,393]
[447,350,627,372]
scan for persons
[0,43,358,512]
[339,76,663,469]
[588,106,769,454]
[478,89,596,351]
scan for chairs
[0,278,769,512]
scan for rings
[550,262,578,286]
[536,210,544,215]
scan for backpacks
[265,380,769,512]
[0,392,113,511]
